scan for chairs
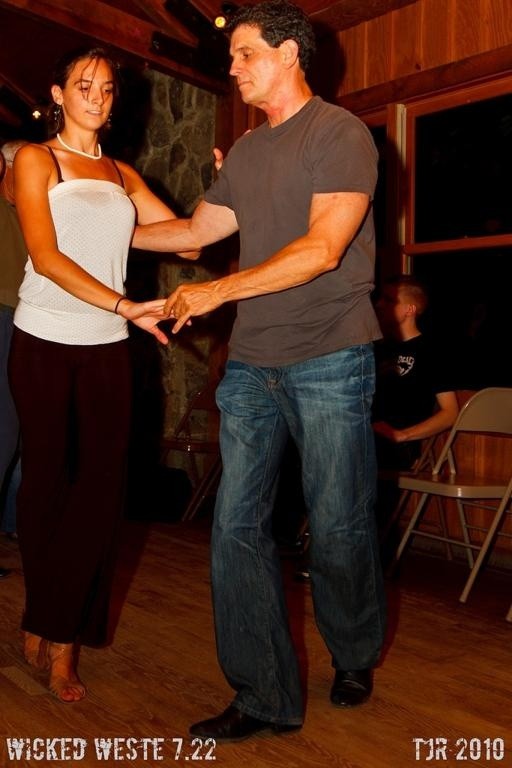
[153,379,230,524]
[391,389,509,601]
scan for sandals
[47,642,88,705]
[23,631,49,671]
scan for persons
[132,0,388,746]
[12,40,252,700]
[0,140,30,540]
[295,274,461,582]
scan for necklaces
[57,132,102,161]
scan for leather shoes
[187,703,304,744]
[330,668,375,708]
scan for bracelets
[114,297,128,315]
